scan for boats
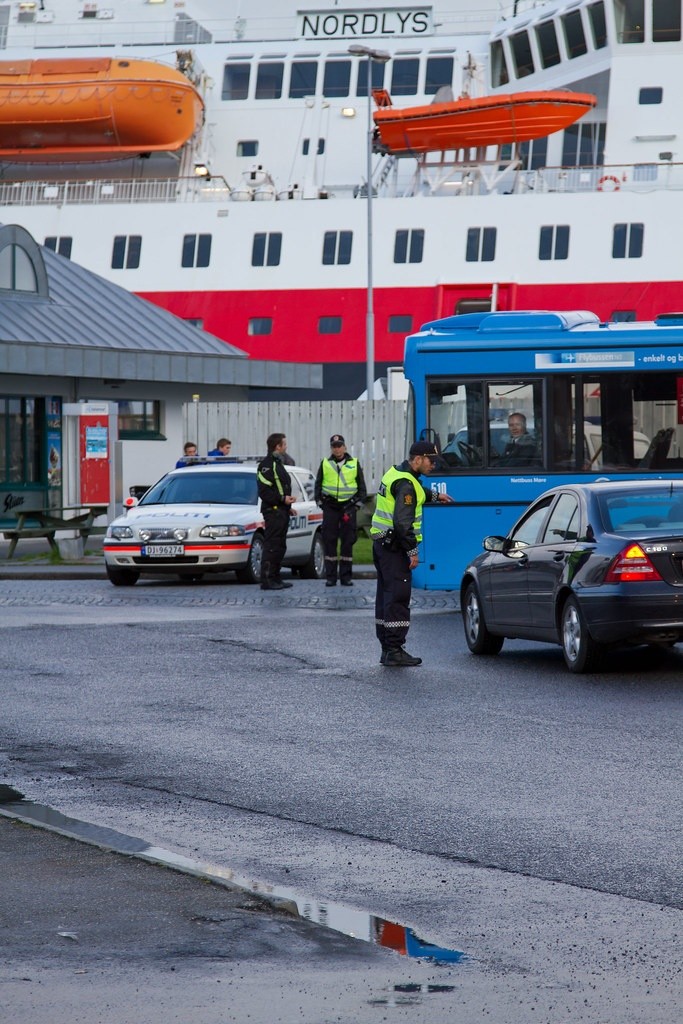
[0,57,207,157]
[376,92,597,154]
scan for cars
[101,455,323,585]
[458,478,682,673]
[440,421,653,470]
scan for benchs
[0,525,109,540]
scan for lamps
[194,159,215,181]
[341,106,357,118]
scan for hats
[409,441,444,462]
[330,434,345,445]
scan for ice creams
[50,447,59,469]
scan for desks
[6,504,135,561]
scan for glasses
[331,443,342,448]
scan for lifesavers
[598,175,620,192]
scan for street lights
[347,46,391,404]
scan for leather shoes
[379,650,386,664]
[384,646,423,665]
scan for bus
[403,310,683,592]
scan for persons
[370,440,454,666]
[490,413,537,467]
[176,442,206,469]
[208,438,241,464]
[256,433,297,590]
[314,436,367,586]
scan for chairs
[613,523,646,531]
[636,427,674,470]
[667,504,683,522]
[657,522,683,528]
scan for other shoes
[325,580,336,587]
[341,578,353,586]
[277,579,293,588]
[261,581,283,589]
